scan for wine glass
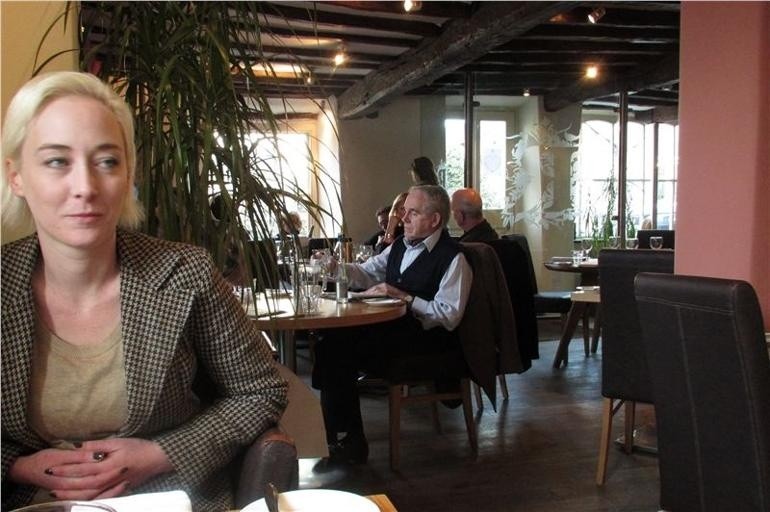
[569,241,593,268]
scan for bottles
[335,238,350,303]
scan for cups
[608,235,621,249]
[311,249,331,279]
[352,244,375,264]
[626,239,639,249]
[295,285,322,314]
[650,236,663,250]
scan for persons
[364,158,440,256]
[311,186,472,471]
[451,188,500,245]
[2,69,290,512]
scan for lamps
[522,88,531,98]
[306,67,316,88]
[404,1,422,14]
[334,41,351,68]
[589,3,605,24]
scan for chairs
[357,243,492,473]
[634,270,770,511]
[305,239,355,292]
[596,248,673,488]
[501,234,589,365]
[471,239,522,408]
[226,431,296,512]
[638,231,674,251]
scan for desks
[229,283,409,385]
[543,255,600,371]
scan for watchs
[405,295,412,301]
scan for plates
[238,487,381,512]
[575,285,599,293]
